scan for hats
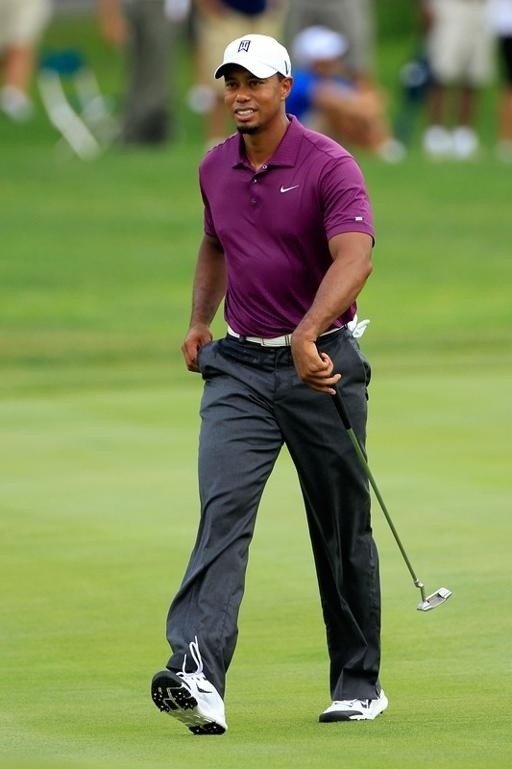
[214,33,291,80]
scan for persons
[150,34,389,734]
[0,0,511,162]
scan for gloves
[347,313,370,339]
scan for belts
[225,326,344,347]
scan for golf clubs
[326,374,452,612]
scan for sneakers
[319,690,388,723]
[150,671,228,734]
